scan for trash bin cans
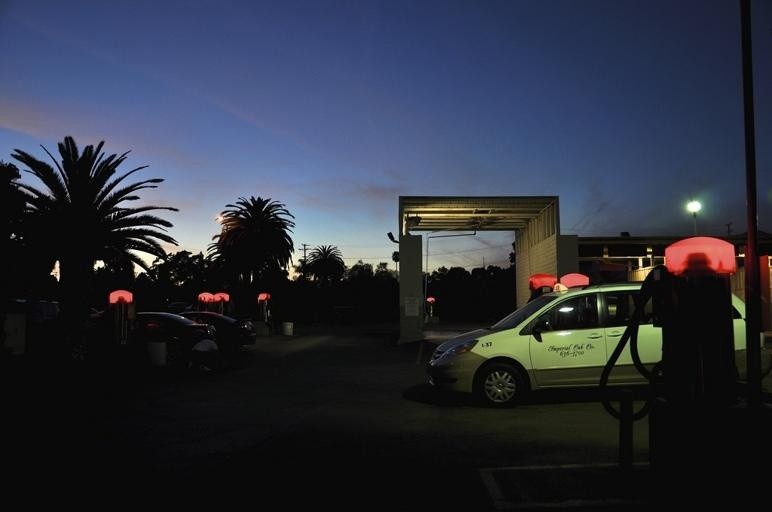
[282,322,294,336]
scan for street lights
[198,289,214,312]
[108,289,134,345]
[255,291,274,321]
[214,292,231,315]
[686,200,703,237]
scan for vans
[423,279,751,409]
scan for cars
[39,310,218,364]
[180,310,258,362]
